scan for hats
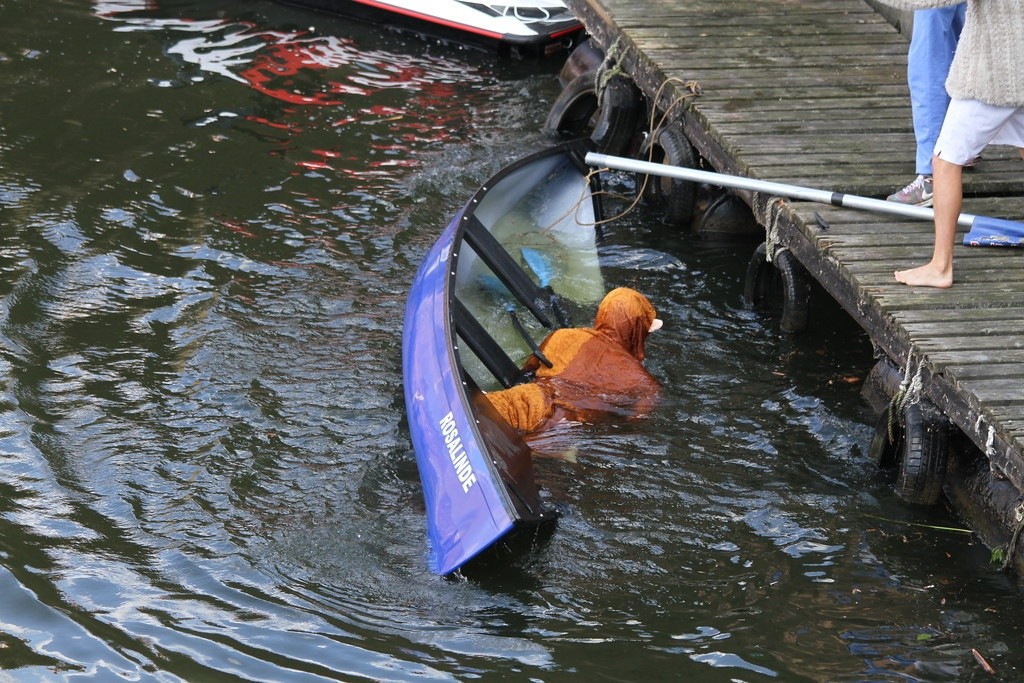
[647,318,663,333]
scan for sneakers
[885,174,934,207]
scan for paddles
[506,309,553,370]
[521,245,569,328]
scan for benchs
[452,291,529,390]
[466,216,567,330]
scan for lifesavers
[870,397,954,509]
[743,239,826,345]
[633,125,699,229]
[543,67,646,160]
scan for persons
[879,0,1024,289]
[886,0,969,208]
[483,288,663,434]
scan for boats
[402,137,607,579]
[274,0,587,64]
